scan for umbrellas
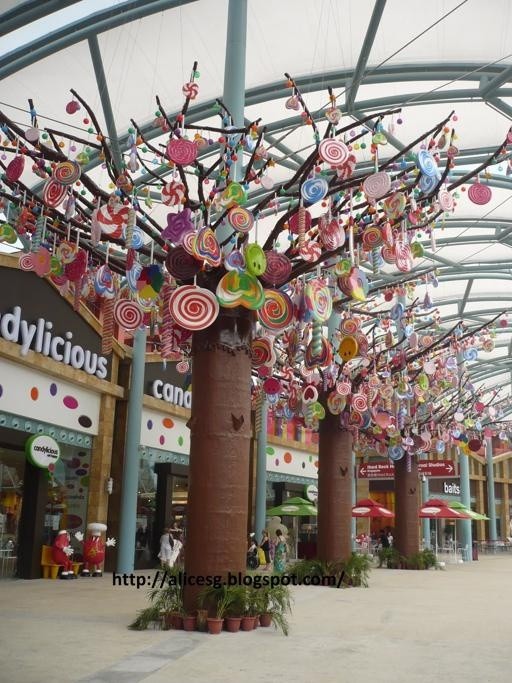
[351,498,395,553]
[266,496,318,559]
[418,497,471,560]
[446,501,490,560]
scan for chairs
[356,533,512,565]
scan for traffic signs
[419,461,460,477]
[357,462,395,478]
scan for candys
[1,83,511,462]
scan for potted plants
[366,544,440,574]
[128,559,291,643]
[282,550,376,596]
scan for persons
[135,528,147,560]
[355,528,393,568]
[157,522,290,572]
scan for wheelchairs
[247,547,260,568]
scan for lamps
[104,477,114,497]
